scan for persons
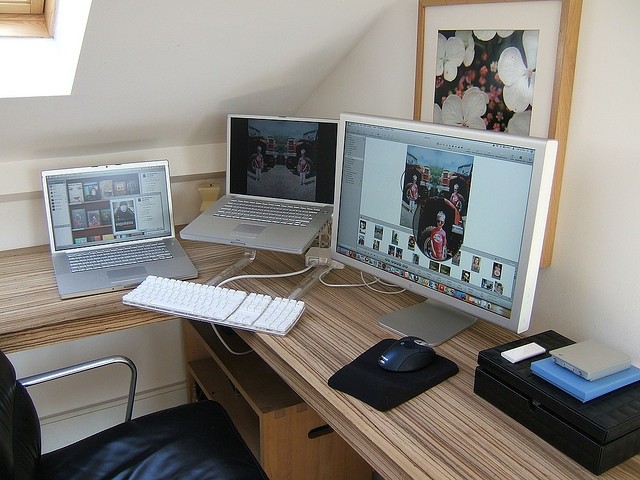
[494,264,501,279]
[406,175,418,214]
[360,222,366,234]
[298,149,310,186]
[494,283,502,294]
[254,146,264,183]
[388,246,394,256]
[450,183,466,223]
[397,249,402,259]
[393,233,398,243]
[452,251,461,266]
[471,257,480,272]
[115,201,134,222]
[430,211,447,259]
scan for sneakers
[258,178,262,181]
[303,183,306,185]
[412,210,415,214]
[255,178,258,181]
[300,181,303,185]
[409,210,411,212]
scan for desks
[0,223,640,476]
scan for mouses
[377,335,437,372]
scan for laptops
[40,159,198,300]
[179,113,341,254]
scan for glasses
[455,188,458,191]
[257,149,261,151]
[301,153,305,155]
[437,219,445,224]
[413,179,416,181]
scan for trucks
[438,164,473,214]
[247,127,279,174]
[283,130,318,178]
[403,153,435,206]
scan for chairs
[0,344,265,474]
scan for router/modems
[304,245,345,270]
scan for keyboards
[122,274,307,338]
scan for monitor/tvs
[330,113,558,349]
